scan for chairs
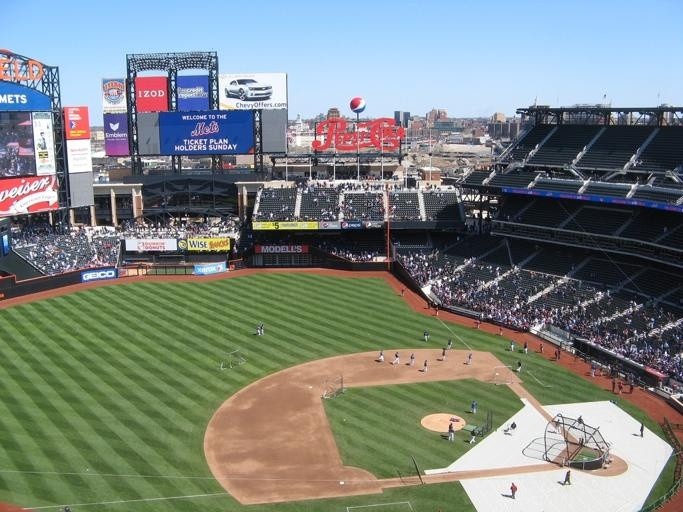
[0,123,682,388]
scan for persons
[562,470,571,485]
[470,401,477,414]
[612,379,616,392]
[12,215,244,279]
[509,482,516,499]
[469,429,477,444]
[244,173,391,263]
[38,131,49,165]
[378,349,384,362]
[448,423,454,442]
[629,380,634,395]
[391,181,683,382]
[510,422,516,435]
[618,381,623,395]
[639,423,643,437]
[1,111,37,177]
[261,324,264,335]
[255,324,261,335]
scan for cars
[225,79,273,101]
